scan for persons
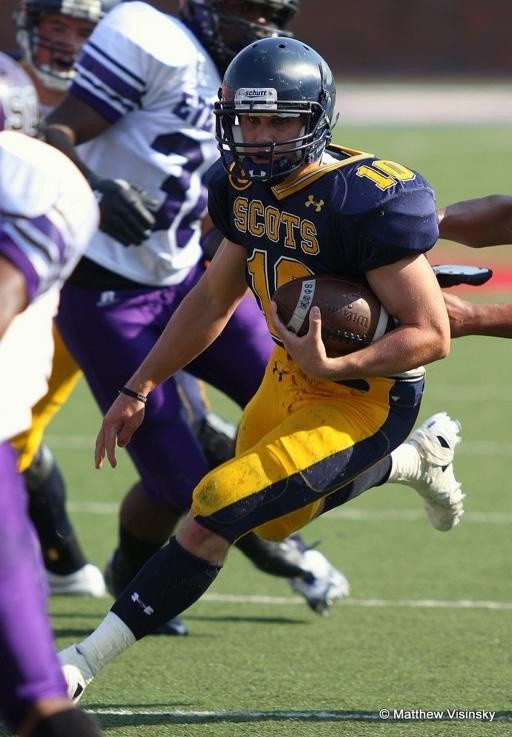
[0,0,512,736]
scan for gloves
[90,177,163,249]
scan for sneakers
[104,550,191,638]
[49,560,107,600]
[55,643,97,706]
[290,547,350,616]
[407,410,465,532]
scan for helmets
[218,33,337,165]
[0,49,42,141]
[16,0,122,57]
[179,0,300,44]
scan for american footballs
[272,274,398,359]
